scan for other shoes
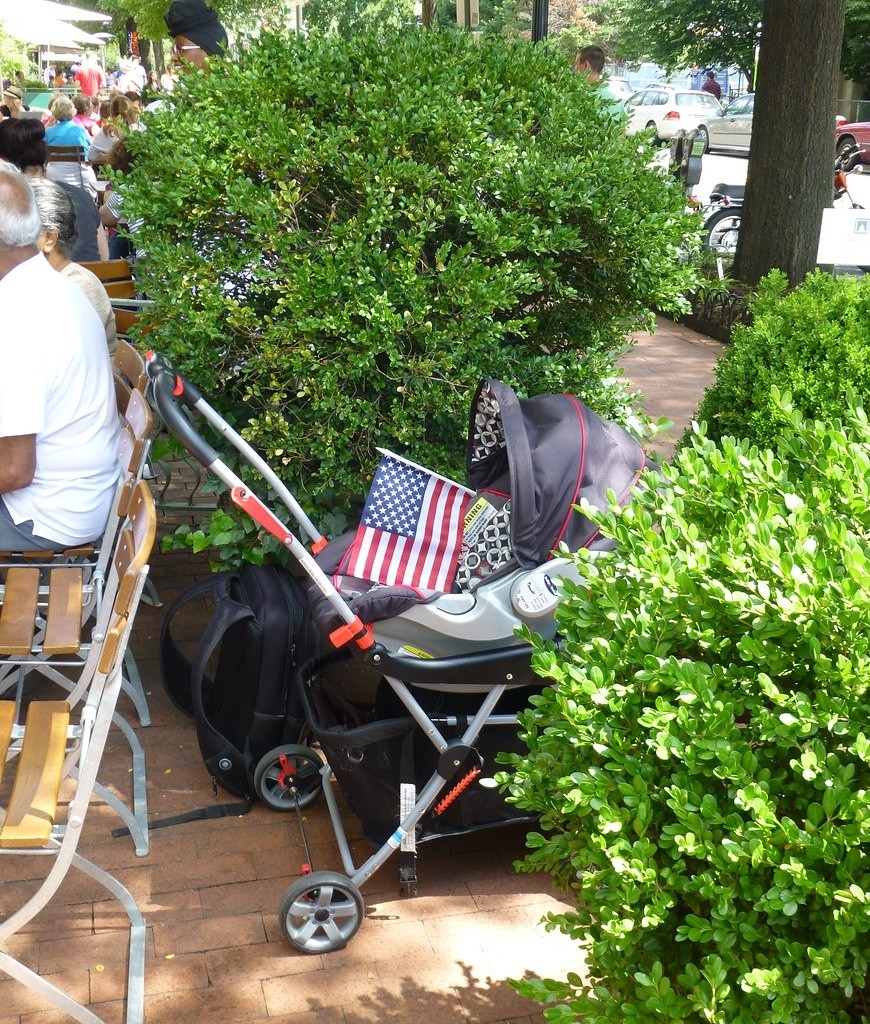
[33,554,91,615]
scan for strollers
[141,344,683,955]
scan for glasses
[169,43,200,58]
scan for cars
[695,92,755,156]
[836,115,870,172]
[618,83,725,148]
[599,61,670,104]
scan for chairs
[0,145,178,1024]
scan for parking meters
[668,127,706,197]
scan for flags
[126,31,139,54]
[347,453,471,601]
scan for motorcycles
[695,142,866,264]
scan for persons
[0,51,180,553]
[702,71,721,101]
[163,0,229,80]
[572,41,627,145]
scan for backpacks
[158,563,314,801]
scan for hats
[2,85,22,100]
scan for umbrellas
[0,0,113,88]
